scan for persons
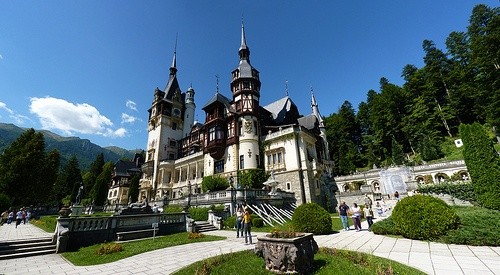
[376,202,384,218]
[233,207,244,238]
[0,207,31,228]
[349,203,362,232]
[338,201,351,231]
[240,206,253,245]
[362,204,374,229]
[363,194,373,209]
[394,191,400,202]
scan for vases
[254,233,320,274]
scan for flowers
[268,227,298,238]
